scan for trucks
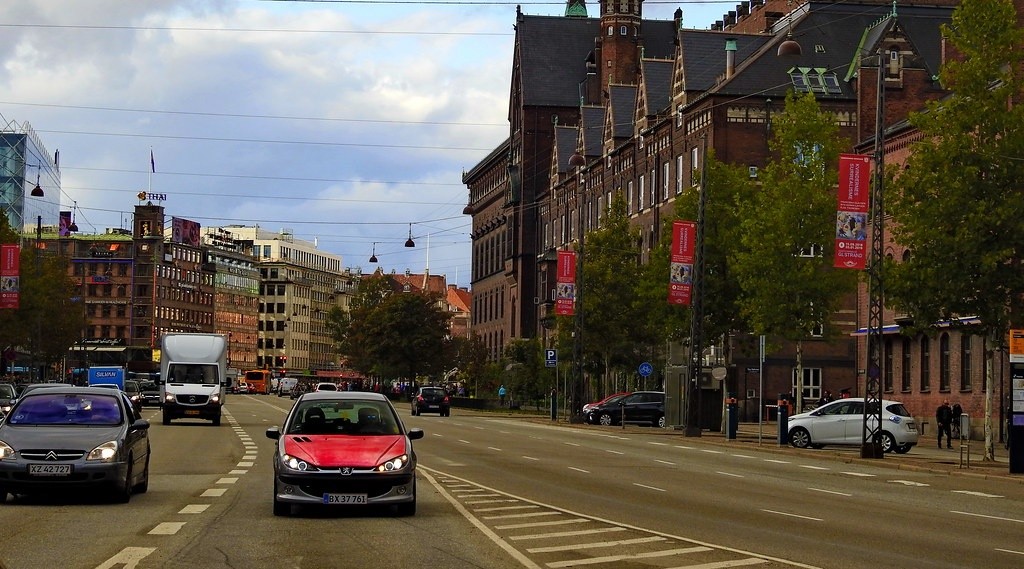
[225,367,237,393]
[159,332,229,425]
[277,378,299,397]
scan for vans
[316,382,338,392]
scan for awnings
[69,346,126,351]
[850,315,980,336]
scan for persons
[394,381,464,397]
[817,390,833,407]
[789,391,805,414]
[498,385,505,406]
[0,373,42,384]
[294,378,380,393]
[360,409,378,425]
[935,399,953,449]
[1004,395,1010,450]
[89,400,117,424]
[951,403,963,439]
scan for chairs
[175,370,185,382]
[358,408,380,424]
[91,400,113,409]
[57,406,68,417]
[640,396,648,402]
[304,407,325,422]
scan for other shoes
[937,443,942,448]
[947,444,953,449]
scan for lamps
[568,126,585,166]
[405,223,415,247]
[31,161,44,196]
[68,207,78,231]
[284,277,352,327]
[370,243,377,262]
[90,232,99,252]
[778,0,802,55]
[463,202,473,215]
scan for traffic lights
[283,356,287,363]
[280,357,283,365]
[280,369,283,377]
[283,370,286,377]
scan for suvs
[290,382,312,400]
[788,398,919,454]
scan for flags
[151,150,155,172]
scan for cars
[0,366,159,504]
[224,381,248,394]
[410,387,451,417]
[582,391,666,428]
[265,391,424,516]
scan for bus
[244,369,274,394]
